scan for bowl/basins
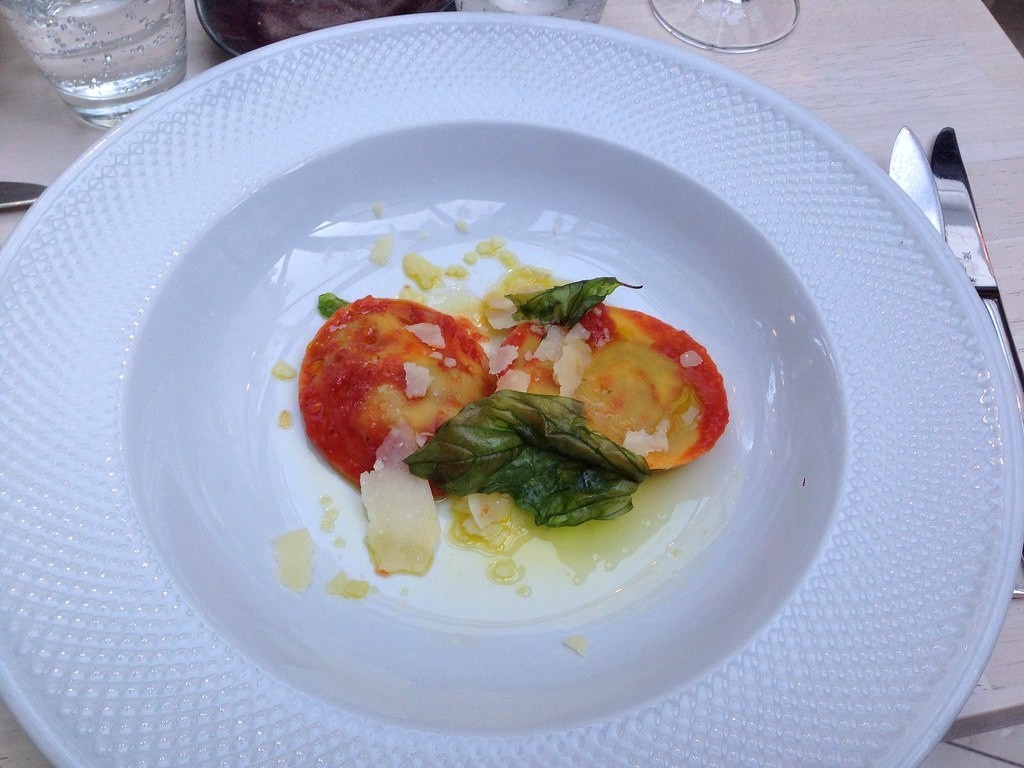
[193,0,452,55]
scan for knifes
[886,127,945,239]
[930,126,1023,416]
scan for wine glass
[649,0,800,53]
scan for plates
[0,9,1023,768]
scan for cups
[1,0,187,128]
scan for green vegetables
[318,277,650,527]
